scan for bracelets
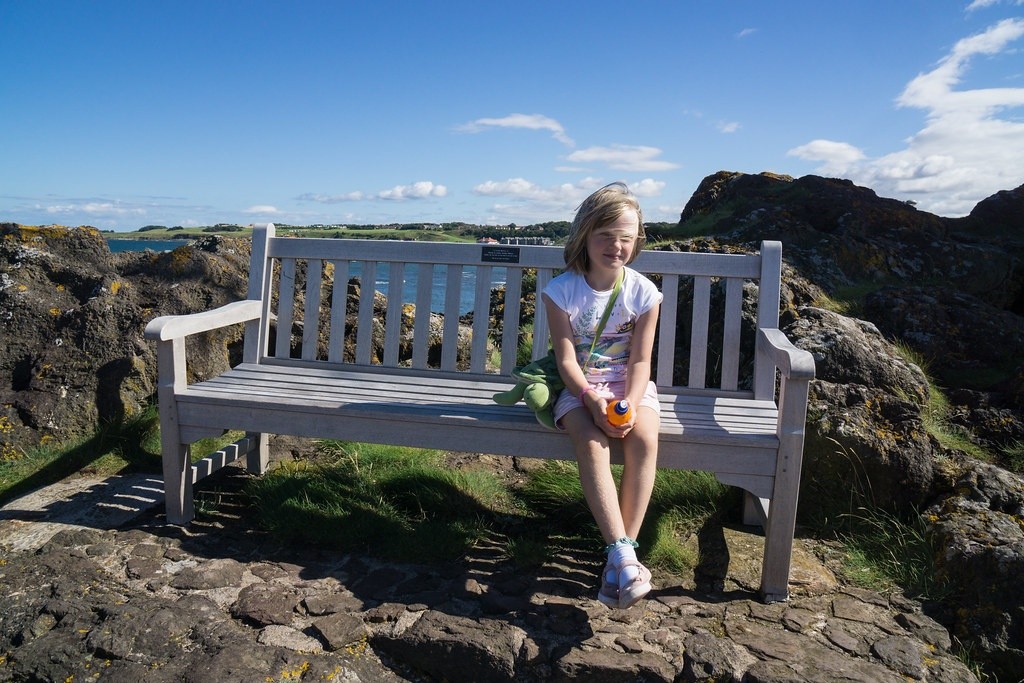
[580,387,594,402]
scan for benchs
[144,222,815,598]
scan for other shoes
[619,560,651,609]
[598,562,619,607]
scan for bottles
[607,399,632,427]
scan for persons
[541,183,663,609]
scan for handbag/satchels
[492,352,566,430]
